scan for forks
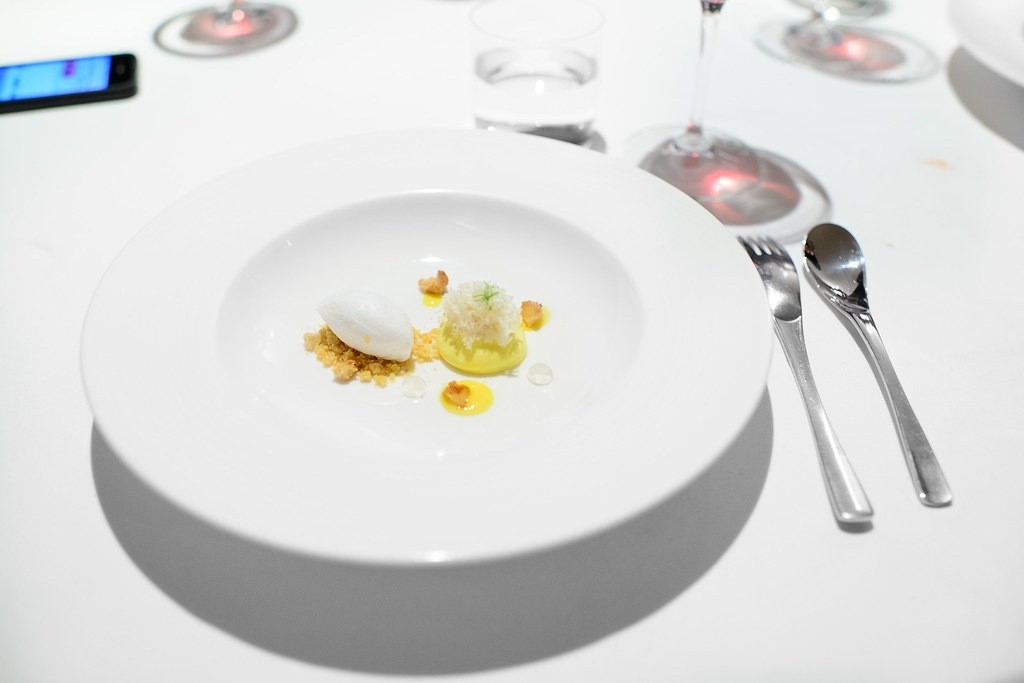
[736,233,875,526]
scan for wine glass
[182,0,281,46]
[779,0,867,63]
[629,0,766,204]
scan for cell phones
[0,53,137,115]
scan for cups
[468,2,608,145]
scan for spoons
[802,221,952,509]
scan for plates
[78,127,780,570]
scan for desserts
[314,280,414,362]
